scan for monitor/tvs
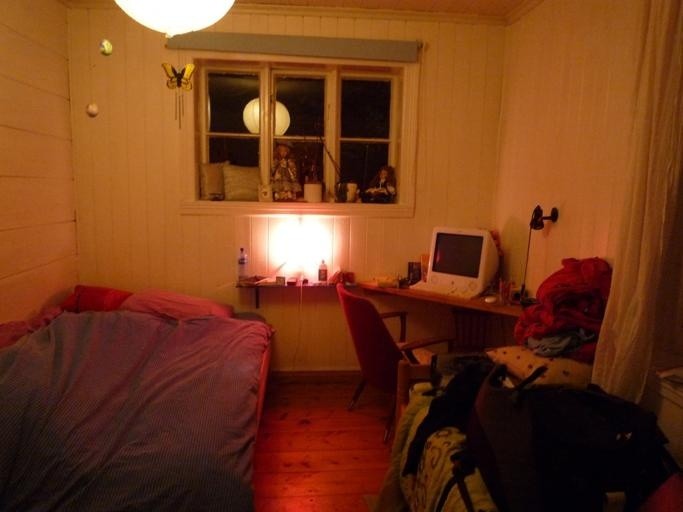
[426,226,500,290]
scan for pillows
[61,284,134,314]
[122,289,235,326]
[486,344,592,389]
[219,162,262,202]
[199,160,230,201]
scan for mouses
[485,295,497,303]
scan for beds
[382,356,681,512]
[0,306,271,511]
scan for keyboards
[410,279,480,301]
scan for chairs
[335,280,459,445]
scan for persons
[363,165,397,203]
[268,141,302,203]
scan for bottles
[318,259,327,283]
[492,238,504,290]
[238,247,248,278]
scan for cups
[420,255,429,282]
[303,183,323,202]
[498,278,512,306]
[346,181,358,202]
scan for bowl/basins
[376,277,393,287]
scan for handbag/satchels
[466,363,667,510]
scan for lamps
[110,0,254,126]
[529,204,559,230]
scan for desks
[358,273,544,327]
